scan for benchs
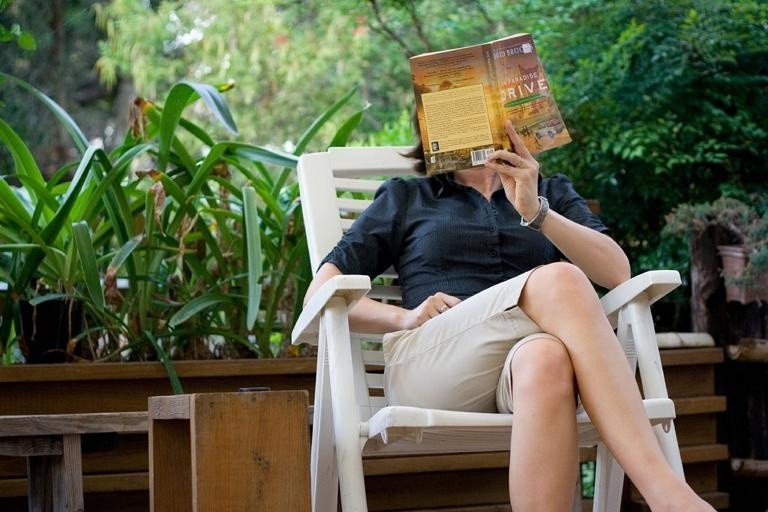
[2,409,147,508]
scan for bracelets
[517,195,550,231]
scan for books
[408,32,573,176]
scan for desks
[147,390,308,512]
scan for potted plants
[664,194,767,307]
[0,78,151,362]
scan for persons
[302,97,717,512]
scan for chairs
[291,141,696,512]
